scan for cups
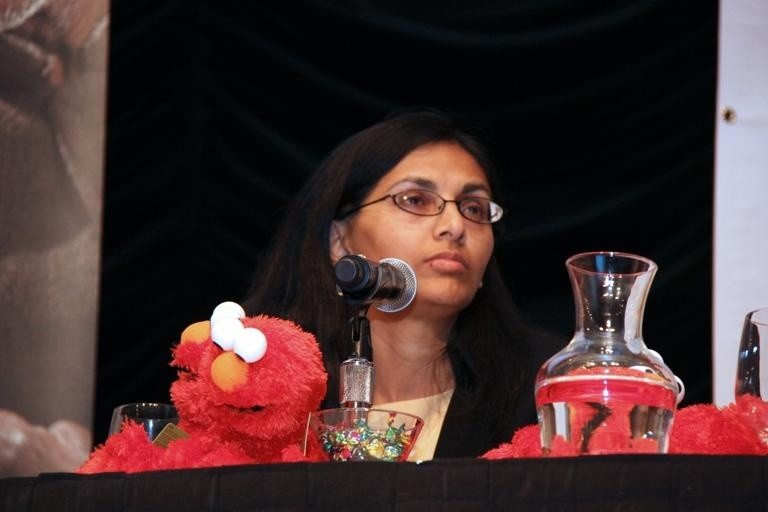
[735,303,768,455]
[109,402,182,444]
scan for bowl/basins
[315,408,425,461]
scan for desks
[0,453,767,511]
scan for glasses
[340,190,507,225]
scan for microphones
[333,253,418,314]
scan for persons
[240,110,545,462]
